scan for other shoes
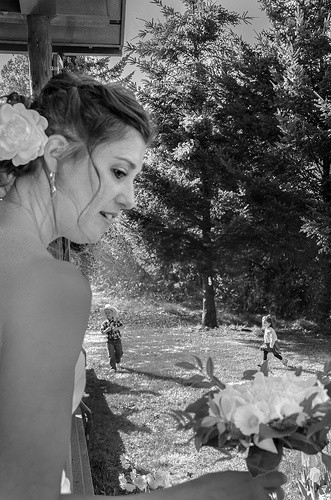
[258,360,270,368]
[108,363,122,374]
[282,359,288,366]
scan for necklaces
[2,198,43,246]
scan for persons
[100,304,124,374]
[258,313,287,369]
[0,72,287,499]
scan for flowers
[0,102,48,166]
[173,353,331,500]
